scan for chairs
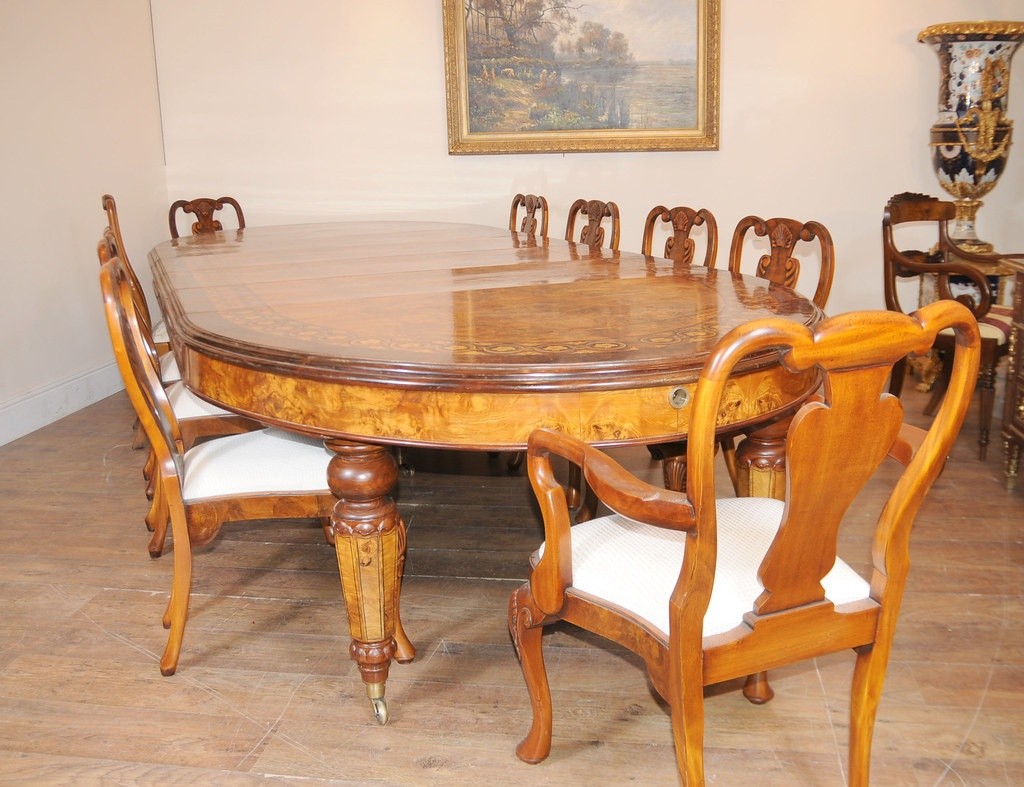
[97,226,334,561]
[506,299,981,787]
[101,192,182,450]
[99,256,414,679]
[488,199,620,459]
[882,190,1024,462]
[168,196,245,239]
[573,216,836,526]
[508,194,549,236]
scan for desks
[148,220,829,726]
[996,258,1024,493]
[909,251,1015,394]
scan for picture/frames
[442,0,719,156]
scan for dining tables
[509,204,718,511]
